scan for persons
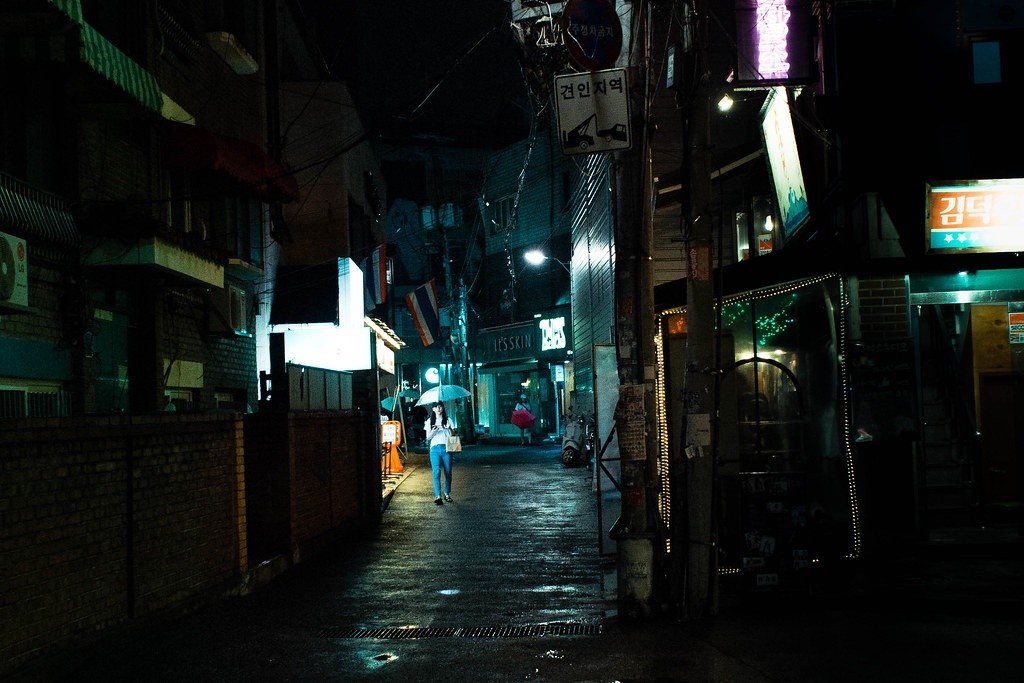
[424,401,455,503]
[397,397,428,448]
[515,394,533,447]
[381,409,393,421]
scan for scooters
[582,412,595,460]
[561,407,587,465]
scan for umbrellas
[398,390,421,398]
[511,410,535,429]
[415,385,472,428]
[381,397,401,411]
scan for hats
[520,394,527,399]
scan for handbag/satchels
[446,427,462,452]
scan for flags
[364,241,386,311]
[405,279,441,346]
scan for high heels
[434,496,442,504]
[443,492,453,503]
